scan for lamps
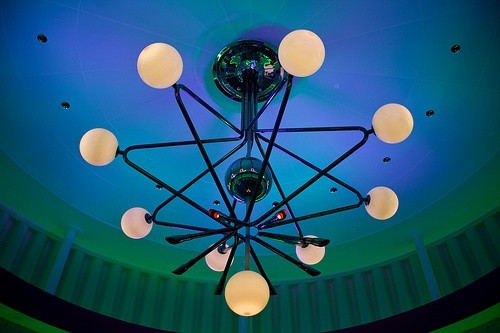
[78,25,417,316]
